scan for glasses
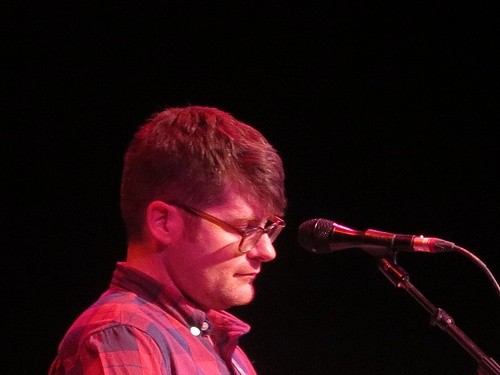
[164,199,286,253]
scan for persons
[48,106,285,374]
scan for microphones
[297,218,454,254]
